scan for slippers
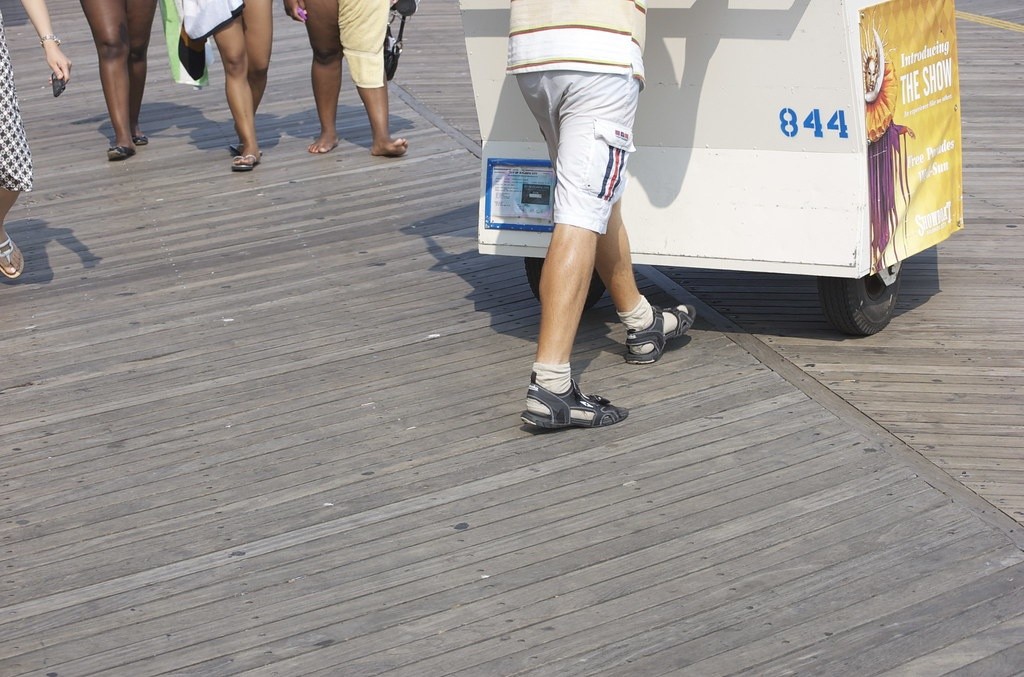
[229,143,263,156]
[231,155,259,170]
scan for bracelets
[39,34,61,47]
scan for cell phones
[297,7,307,20]
[52,67,66,98]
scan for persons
[79,0,157,161]
[178,0,273,171]
[506,0,695,428]
[284,0,410,157]
[0,0,72,279]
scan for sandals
[521,372,629,432]
[0,232,24,279]
[623,304,697,364]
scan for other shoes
[108,146,136,159]
[132,134,148,145]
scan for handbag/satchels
[178,24,207,81]
[384,13,406,81]
